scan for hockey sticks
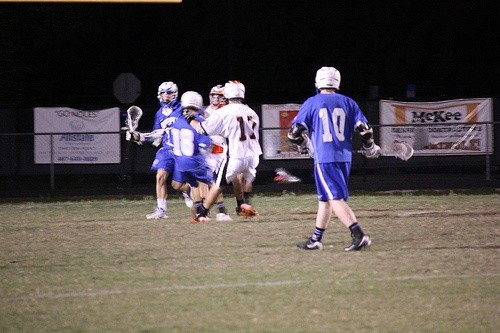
[278,142,414,162]
[120,105,144,177]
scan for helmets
[209,85,229,105]
[315,66,341,90]
[181,91,203,111]
[157,81,179,107]
[224,80,245,99]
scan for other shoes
[190,213,209,224]
[235,203,260,217]
[216,213,233,222]
[182,184,195,208]
[145,207,169,221]
[191,205,203,217]
[296,240,323,250]
[344,235,372,253]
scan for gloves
[126,131,145,145]
[297,139,311,157]
[182,109,196,124]
[363,142,381,161]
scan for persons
[287,67,382,251]
[183,80,263,222]
[162,91,233,221]
[125,81,183,219]
